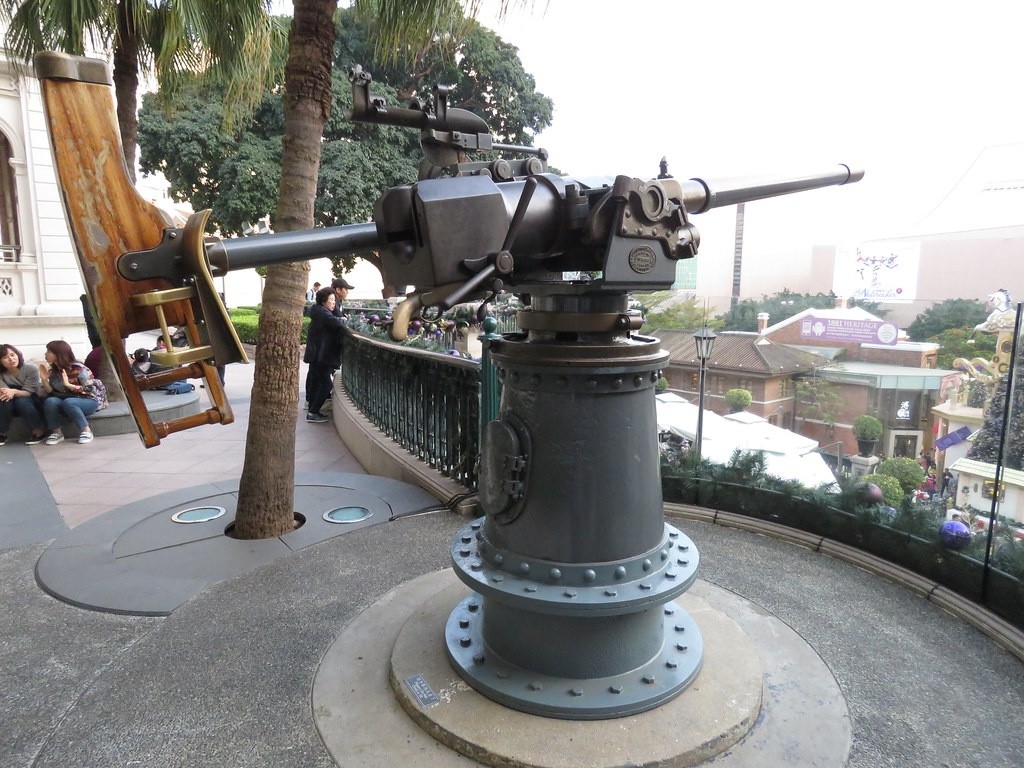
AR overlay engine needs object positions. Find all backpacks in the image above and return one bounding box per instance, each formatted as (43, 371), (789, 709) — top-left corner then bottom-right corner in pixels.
(67, 362), (109, 411)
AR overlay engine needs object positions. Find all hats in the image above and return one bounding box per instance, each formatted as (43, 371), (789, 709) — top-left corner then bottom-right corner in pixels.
(331, 278), (355, 289)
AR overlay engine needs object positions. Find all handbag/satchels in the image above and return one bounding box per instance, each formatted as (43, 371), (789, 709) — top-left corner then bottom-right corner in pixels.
(167, 381), (195, 395)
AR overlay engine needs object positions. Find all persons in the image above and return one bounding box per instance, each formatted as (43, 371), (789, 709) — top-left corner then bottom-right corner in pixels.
(39, 340), (103, 444)
(302, 279), (355, 409)
(0, 344), (50, 446)
(917, 450), (953, 503)
(305, 282), (321, 301)
(304, 287), (348, 422)
(80, 293), (227, 401)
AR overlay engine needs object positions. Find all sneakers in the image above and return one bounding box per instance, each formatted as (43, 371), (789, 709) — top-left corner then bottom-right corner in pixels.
(45, 432), (64, 444)
(77, 431), (94, 443)
(0, 434), (8, 445)
(306, 410), (329, 423)
(24, 431), (48, 445)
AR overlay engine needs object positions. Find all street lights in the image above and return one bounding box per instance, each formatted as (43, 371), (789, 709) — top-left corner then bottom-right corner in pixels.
(692, 326), (717, 460)
